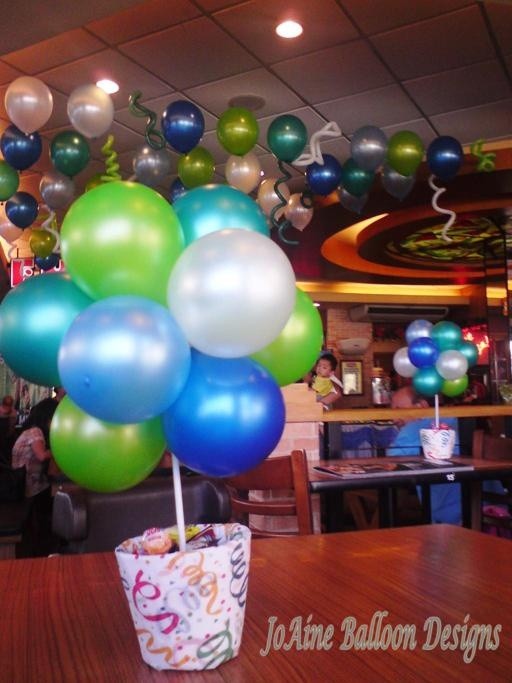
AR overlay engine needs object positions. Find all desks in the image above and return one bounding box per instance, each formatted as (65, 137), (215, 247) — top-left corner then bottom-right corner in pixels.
(307, 453), (512, 531)
(0, 523), (512, 682)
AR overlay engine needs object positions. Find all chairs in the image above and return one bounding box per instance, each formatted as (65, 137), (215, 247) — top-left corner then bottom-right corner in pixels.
(50, 476), (231, 554)
(473, 428), (512, 539)
(222, 448), (314, 538)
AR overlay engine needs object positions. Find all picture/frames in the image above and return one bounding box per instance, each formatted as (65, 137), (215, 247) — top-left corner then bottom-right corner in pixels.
(340, 359), (364, 396)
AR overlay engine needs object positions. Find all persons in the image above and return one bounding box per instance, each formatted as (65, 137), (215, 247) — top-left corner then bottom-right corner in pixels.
(310, 350), (346, 439)
(303, 351), (347, 460)
(53, 386), (67, 402)
(10, 396), (63, 553)
(460, 376), (492, 430)
(370, 384), (509, 530)
(0, 392), (20, 431)
(246, 365), (329, 536)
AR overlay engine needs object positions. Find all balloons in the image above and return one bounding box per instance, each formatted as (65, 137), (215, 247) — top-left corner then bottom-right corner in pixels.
(158, 347), (286, 480)
(166, 225), (298, 360)
(0, 273), (96, 390)
(392, 317), (479, 399)
(60, 178), (183, 305)
(54, 294), (191, 425)
(3, 70), (468, 275)
(248, 286), (327, 389)
(47, 393), (169, 494)
